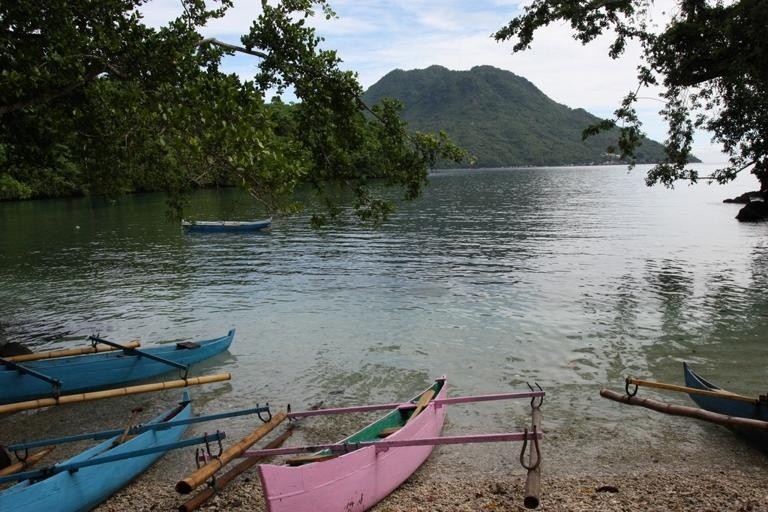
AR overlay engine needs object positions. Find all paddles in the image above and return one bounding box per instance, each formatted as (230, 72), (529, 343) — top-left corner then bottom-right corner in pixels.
(407, 389), (435, 422)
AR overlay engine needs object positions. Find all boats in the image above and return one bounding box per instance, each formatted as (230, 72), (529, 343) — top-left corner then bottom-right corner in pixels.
(181, 217), (273, 229)
(183, 227), (274, 238)
(0, 327), (235, 402)
(257, 373), (452, 511)
(683, 360), (768, 445)
(0, 389), (196, 512)
